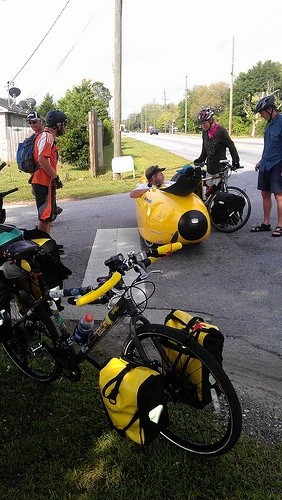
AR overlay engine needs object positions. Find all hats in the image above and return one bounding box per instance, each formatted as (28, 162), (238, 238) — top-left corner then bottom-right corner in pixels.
(26, 112), (41, 122)
(145, 165), (166, 179)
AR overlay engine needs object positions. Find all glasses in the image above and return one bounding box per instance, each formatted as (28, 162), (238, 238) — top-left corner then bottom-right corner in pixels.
(28, 120), (38, 125)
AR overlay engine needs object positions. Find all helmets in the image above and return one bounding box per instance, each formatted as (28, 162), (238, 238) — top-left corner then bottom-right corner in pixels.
(256, 94), (275, 112)
(197, 108), (215, 122)
(46, 110), (68, 126)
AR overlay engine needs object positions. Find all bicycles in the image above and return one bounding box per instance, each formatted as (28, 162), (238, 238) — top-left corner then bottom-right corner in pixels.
(174, 159), (252, 233)
(1, 239), (243, 460)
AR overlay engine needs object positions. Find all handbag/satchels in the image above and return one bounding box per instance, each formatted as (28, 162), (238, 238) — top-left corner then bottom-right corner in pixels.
(160, 309), (223, 410)
(17, 230), (71, 319)
(98, 358), (172, 446)
(213, 192), (246, 226)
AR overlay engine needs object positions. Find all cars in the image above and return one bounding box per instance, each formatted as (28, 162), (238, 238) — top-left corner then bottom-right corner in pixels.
(149, 128), (158, 135)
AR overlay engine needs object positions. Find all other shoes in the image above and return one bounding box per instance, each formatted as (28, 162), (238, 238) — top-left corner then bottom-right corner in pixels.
(50, 206), (63, 221)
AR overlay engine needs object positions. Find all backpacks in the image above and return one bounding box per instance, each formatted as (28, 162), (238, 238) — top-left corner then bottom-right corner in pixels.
(17, 130), (54, 173)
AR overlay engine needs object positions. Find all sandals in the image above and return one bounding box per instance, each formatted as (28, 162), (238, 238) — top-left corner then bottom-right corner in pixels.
(250, 222), (271, 231)
(272, 226), (282, 236)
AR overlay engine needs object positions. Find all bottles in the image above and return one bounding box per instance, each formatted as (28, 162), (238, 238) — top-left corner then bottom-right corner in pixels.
(205, 183), (216, 197)
(202, 184), (207, 201)
(73, 314), (94, 344)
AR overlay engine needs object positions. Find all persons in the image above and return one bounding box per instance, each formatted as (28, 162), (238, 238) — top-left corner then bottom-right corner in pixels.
(129, 166), (177, 198)
(194, 108), (240, 228)
(251, 95), (282, 237)
(26, 109), (68, 254)
